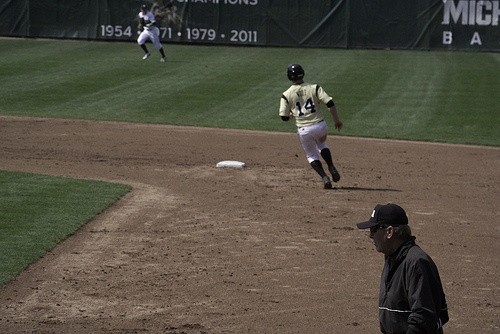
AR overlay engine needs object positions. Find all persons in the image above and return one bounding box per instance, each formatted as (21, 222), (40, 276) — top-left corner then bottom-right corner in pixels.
(357, 202), (449, 334)
(137, 4), (166, 63)
(279, 64), (343, 189)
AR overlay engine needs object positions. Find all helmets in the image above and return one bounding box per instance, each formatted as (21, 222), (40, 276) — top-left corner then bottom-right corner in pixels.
(287, 64), (304, 80)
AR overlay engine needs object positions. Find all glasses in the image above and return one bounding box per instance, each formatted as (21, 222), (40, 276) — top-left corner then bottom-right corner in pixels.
(370, 226), (400, 234)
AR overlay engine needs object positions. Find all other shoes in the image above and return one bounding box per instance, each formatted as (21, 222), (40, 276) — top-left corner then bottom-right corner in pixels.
(323, 176), (332, 189)
(142, 53), (151, 60)
(331, 169), (340, 183)
(160, 57), (166, 62)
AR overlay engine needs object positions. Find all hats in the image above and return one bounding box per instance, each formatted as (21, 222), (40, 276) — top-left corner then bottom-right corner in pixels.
(140, 5), (147, 8)
(357, 202), (408, 229)
